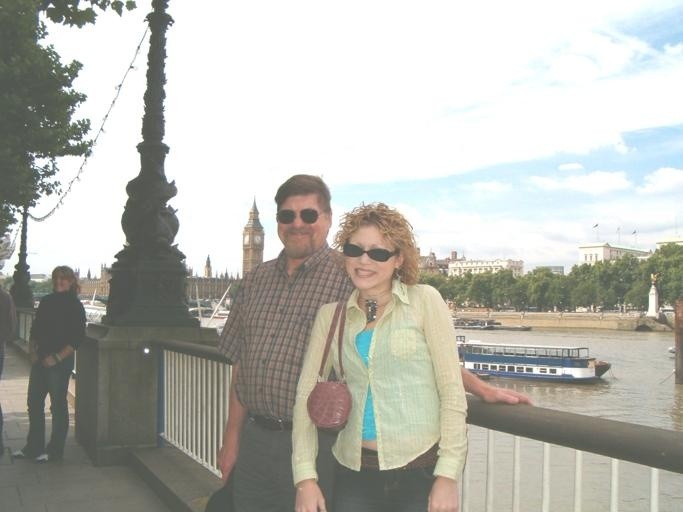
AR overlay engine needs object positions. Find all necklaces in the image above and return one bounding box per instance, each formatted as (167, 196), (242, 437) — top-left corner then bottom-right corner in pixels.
(358, 290), (393, 322)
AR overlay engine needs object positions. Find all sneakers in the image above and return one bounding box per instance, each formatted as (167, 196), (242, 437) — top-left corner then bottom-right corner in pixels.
(11, 441), (63, 463)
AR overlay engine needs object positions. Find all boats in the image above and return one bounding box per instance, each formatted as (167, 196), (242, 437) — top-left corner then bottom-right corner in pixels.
(456, 335), (611, 383)
(453, 317), (532, 331)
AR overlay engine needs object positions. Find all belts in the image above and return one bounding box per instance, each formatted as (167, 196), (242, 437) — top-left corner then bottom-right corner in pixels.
(256, 418), (293, 431)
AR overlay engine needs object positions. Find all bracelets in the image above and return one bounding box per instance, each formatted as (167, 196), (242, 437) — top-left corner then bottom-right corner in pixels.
(53, 353), (63, 363)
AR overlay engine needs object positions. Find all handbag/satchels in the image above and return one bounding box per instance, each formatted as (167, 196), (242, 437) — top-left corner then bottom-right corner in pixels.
(307, 380), (352, 430)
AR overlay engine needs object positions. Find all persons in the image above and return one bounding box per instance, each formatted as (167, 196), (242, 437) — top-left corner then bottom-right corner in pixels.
(0, 289), (18, 456)
(204, 176), (533, 511)
(14, 264), (87, 462)
(288, 201), (470, 511)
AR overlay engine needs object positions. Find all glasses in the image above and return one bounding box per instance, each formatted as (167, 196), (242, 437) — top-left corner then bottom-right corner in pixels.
(276, 209), (318, 224)
(343, 238), (396, 261)
